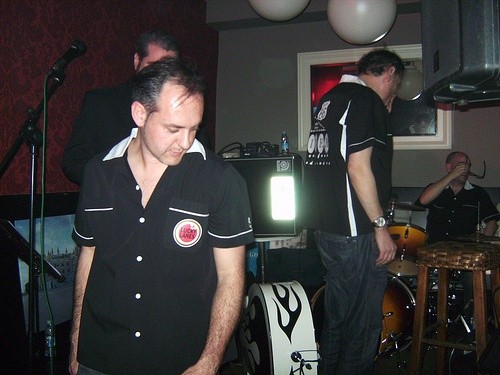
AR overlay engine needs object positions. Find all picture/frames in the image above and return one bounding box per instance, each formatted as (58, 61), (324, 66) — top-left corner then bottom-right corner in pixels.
(296, 44), (452, 155)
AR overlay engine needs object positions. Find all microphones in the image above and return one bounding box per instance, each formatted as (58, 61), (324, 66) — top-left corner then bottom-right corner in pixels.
(46, 37), (89, 78)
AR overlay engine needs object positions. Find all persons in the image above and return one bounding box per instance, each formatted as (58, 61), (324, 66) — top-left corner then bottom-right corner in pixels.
(413, 151), (500, 324)
(68, 58), (255, 375)
(402, 112), (432, 135)
(61, 30), (180, 185)
(301, 49), (406, 375)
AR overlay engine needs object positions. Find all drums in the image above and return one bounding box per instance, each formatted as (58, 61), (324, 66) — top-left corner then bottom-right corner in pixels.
(312, 274), (417, 360)
(385, 222), (428, 277)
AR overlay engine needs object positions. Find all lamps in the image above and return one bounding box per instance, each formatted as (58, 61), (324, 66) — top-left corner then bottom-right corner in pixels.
(249, 0), (309, 21)
(327, 0), (397, 45)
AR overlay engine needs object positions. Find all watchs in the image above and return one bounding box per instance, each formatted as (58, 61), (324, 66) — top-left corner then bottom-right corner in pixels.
(372, 216), (386, 228)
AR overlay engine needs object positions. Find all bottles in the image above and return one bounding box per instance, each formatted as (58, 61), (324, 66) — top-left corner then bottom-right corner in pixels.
(44, 319), (57, 359)
(281, 132), (289, 156)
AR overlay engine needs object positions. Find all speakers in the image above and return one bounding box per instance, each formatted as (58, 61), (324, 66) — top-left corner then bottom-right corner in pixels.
(220, 154), (305, 236)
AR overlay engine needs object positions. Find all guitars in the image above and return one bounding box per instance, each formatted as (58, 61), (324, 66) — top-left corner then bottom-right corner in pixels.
(237, 281), (318, 375)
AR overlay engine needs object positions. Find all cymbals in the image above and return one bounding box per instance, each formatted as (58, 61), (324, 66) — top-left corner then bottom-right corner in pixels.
(388, 201), (426, 210)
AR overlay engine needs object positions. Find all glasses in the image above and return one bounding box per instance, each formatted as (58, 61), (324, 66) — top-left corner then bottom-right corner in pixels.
(465, 156), (487, 179)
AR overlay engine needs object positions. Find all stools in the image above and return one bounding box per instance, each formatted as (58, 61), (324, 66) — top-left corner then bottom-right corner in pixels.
(409, 239), (500, 375)
